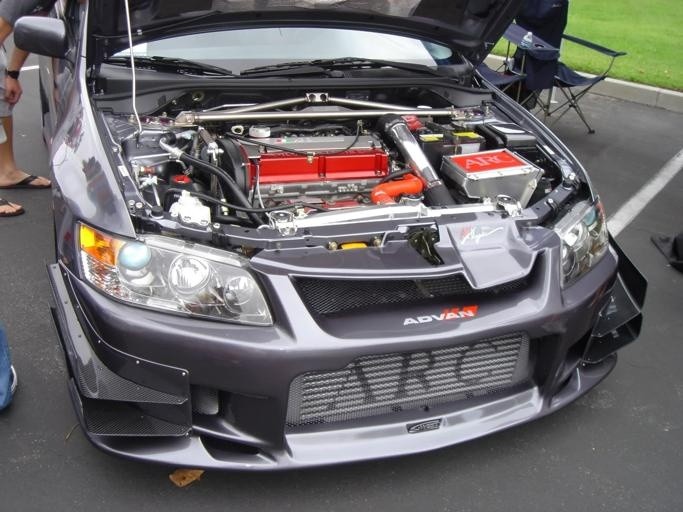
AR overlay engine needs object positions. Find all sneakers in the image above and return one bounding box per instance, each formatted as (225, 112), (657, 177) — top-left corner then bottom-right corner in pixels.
(10, 365), (18, 395)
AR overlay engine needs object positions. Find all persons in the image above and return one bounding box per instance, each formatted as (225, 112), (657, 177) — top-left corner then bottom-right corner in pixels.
(0, 320), (19, 413)
(0, 0), (58, 218)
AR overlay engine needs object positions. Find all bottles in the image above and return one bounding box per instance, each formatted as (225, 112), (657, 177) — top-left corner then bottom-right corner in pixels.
(521, 32), (534, 48)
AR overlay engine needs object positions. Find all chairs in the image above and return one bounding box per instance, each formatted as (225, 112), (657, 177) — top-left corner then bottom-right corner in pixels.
(476, 0), (627, 134)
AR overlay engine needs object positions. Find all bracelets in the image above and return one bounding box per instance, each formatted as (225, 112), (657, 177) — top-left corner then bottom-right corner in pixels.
(4, 68), (21, 80)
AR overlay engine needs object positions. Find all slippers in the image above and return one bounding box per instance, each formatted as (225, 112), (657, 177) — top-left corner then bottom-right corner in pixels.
(0, 176), (51, 189)
(0, 199), (25, 217)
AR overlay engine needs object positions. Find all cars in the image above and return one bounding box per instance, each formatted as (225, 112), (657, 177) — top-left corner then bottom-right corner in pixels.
(14, 0), (649, 472)
